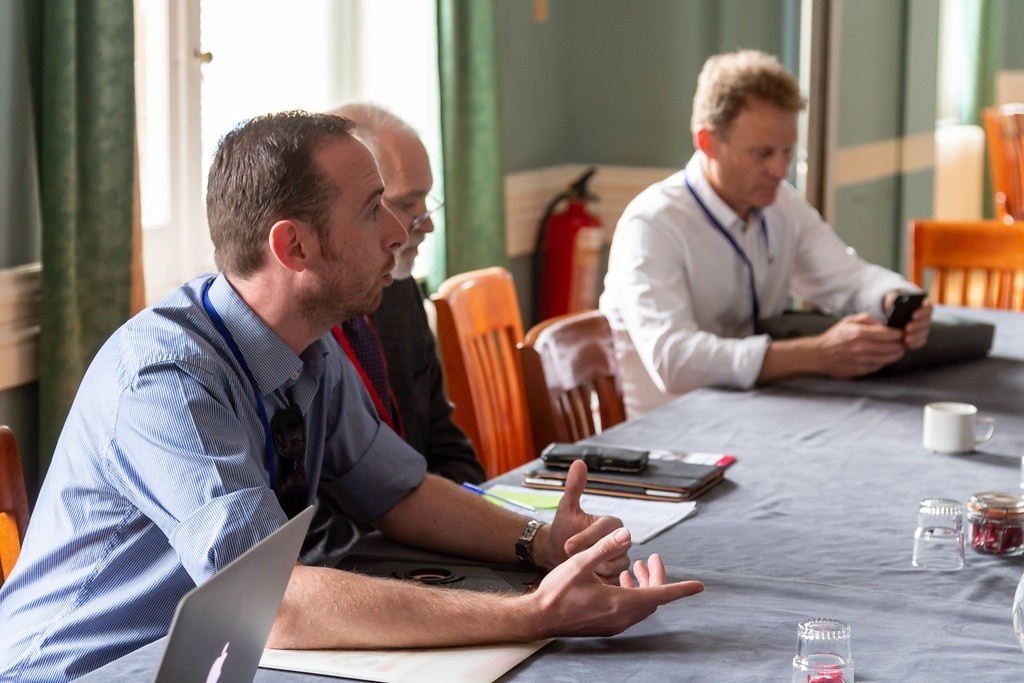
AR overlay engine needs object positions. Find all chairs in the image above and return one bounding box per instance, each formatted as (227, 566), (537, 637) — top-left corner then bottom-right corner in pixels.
(432, 269), (533, 482)
(517, 311), (628, 455)
(985, 99), (1024, 221)
(910, 218), (1024, 313)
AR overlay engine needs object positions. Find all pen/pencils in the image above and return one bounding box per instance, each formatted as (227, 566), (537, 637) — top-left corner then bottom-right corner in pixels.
(463, 481), (535, 513)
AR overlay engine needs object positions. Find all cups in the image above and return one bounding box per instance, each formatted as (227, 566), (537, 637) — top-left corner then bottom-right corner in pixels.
(923, 402), (996, 454)
(911, 499), (966, 573)
(965, 492), (1024, 558)
(790, 620), (855, 683)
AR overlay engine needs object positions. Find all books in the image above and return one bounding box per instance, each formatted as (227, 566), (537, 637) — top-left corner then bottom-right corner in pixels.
(517, 436), (726, 503)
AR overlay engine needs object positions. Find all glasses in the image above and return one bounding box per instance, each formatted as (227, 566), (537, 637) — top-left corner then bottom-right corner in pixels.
(270, 402), (311, 520)
(383, 193), (446, 232)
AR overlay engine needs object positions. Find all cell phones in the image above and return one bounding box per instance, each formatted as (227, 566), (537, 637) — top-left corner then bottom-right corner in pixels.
(884, 293), (926, 329)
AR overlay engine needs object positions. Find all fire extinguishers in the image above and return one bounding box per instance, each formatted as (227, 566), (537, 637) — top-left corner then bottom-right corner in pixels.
(527, 165), (605, 324)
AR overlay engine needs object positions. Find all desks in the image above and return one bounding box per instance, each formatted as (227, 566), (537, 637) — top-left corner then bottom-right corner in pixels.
(71, 305), (1024, 683)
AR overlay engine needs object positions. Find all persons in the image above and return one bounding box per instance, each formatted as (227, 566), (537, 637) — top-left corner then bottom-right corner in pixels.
(595, 46), (934, 417)
(339, 106), (485, 482)
(0, 111), (709, 683)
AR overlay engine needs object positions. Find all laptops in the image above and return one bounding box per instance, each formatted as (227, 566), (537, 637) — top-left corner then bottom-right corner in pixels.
(153, 505), (316, 683)
